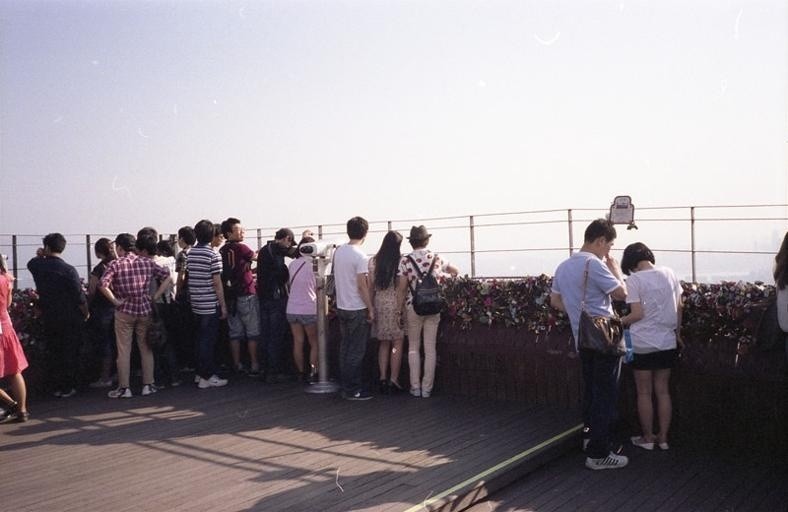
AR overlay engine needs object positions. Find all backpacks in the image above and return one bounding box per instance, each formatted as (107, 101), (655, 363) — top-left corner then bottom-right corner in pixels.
(223, 267), (247, 297)
(404, 253), (442, 316)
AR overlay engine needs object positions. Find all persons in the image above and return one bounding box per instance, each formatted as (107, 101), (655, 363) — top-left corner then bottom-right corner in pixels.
(80, 217), (319, 397)
(615, 241), (682, 455)
(1, 257), (29, 423)
(774, 229), (788, 352)
(332, 216), (458, 400)
(28, 232), (90, 397)
(548, 217), (628, 473)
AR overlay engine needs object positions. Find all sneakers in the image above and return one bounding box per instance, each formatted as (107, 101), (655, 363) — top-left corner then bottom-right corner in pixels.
(422, 390), (431, 397)
(409, 389), (421, 397)
(108, 387), (132, 398)
(194, 375), (228, 389)
(585, 451), (628, 470)
(142, 384), (158, 396)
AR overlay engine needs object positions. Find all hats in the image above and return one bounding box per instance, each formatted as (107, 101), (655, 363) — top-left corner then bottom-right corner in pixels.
(406, 225), (432, 240)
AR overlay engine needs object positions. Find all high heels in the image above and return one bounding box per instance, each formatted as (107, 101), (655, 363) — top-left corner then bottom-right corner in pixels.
(379, 379), (406, 396)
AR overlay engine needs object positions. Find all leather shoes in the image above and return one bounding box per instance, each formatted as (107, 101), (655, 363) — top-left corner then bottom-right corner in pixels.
(631, 436), (669, 450)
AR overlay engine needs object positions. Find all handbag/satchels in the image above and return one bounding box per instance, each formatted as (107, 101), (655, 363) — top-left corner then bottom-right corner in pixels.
(146, 320), (168, 349)
(577, 310), (627, 357)
(326, 274), (335, 297)
